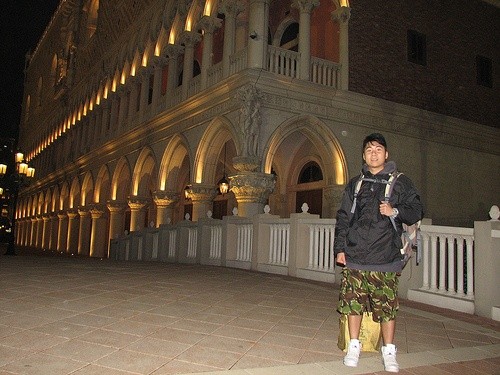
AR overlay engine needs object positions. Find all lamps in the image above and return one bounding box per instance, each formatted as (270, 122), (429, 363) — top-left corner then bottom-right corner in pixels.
(218, 143), (229, 195)
(184, 164), (191, 200)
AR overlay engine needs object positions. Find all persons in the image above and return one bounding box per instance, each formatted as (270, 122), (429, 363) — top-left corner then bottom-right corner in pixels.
(334, 133), (423, 373)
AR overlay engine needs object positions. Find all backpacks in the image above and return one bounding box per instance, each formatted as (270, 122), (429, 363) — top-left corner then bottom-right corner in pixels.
(350, 170), (422, 276)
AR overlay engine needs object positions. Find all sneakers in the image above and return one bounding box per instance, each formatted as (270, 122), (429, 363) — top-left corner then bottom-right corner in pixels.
(381, 344), (399, 373)
(344, 339), (362, 367)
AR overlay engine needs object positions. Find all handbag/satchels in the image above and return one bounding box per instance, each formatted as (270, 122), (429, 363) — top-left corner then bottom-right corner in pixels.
(337, 305), (381, 354)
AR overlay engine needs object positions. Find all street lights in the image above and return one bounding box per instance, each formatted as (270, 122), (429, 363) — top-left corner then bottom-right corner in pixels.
(0, 146), (37, 256)
